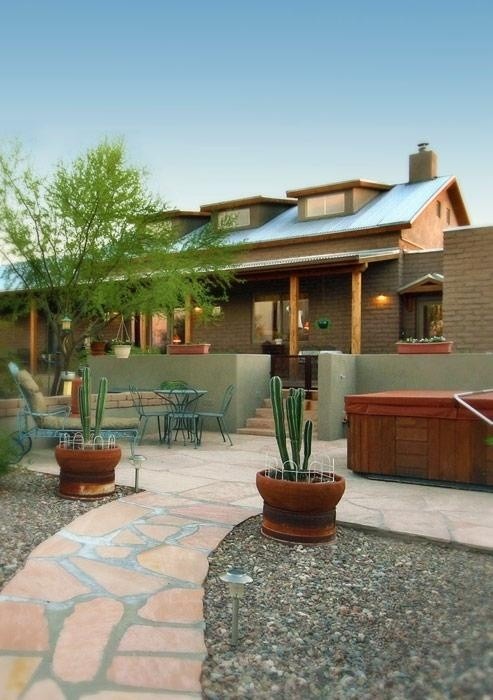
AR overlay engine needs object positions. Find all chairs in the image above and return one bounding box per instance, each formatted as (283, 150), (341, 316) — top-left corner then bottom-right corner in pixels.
(8, 358), (139, 467)
(127, 378), (237, 449)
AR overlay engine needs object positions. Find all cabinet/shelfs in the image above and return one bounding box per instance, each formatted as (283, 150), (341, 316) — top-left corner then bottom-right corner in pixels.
(342, 388), (492, 487)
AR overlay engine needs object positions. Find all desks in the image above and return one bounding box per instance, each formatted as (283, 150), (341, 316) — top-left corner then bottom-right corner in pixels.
(288, 351), (343, 397)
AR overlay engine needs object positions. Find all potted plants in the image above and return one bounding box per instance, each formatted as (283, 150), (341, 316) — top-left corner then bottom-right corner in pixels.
(165, 342), (211, 354)
(255, 373), (347, 546)
(111, 337), (133, 359)
(51, 364), (123, 502)
(394, 335), (456, 355)
(90, 334), (109, 355)
